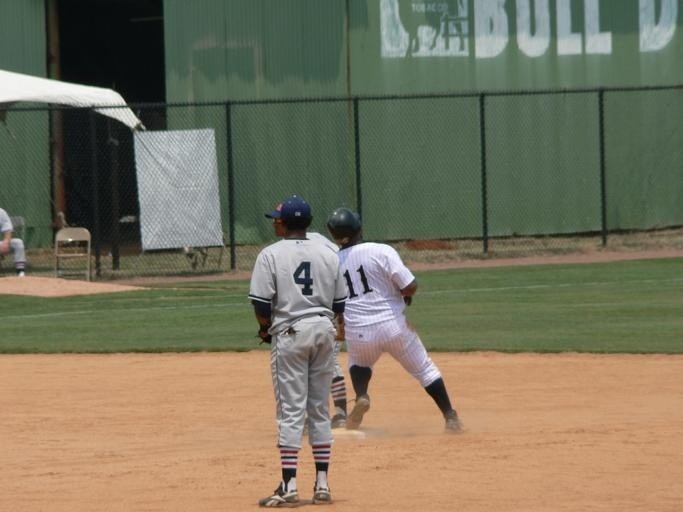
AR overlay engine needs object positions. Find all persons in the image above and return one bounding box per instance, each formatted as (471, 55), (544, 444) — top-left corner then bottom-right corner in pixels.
(264, 204), (347, 436)
(248, 197), (347, 508)
(0, 208), (27, 277)
(327, 207), (467, 434)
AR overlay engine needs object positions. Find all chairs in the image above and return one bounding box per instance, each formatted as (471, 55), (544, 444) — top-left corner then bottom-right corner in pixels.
(54, 227), (91, 282)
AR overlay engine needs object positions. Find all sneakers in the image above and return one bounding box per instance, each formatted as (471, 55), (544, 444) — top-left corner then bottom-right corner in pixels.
(330, 414), (346, 428)
(347, 394), (370, 428)
(313, 482), (331, 504)
(260, 485), (299, 507)
(446, 419), (463, 433)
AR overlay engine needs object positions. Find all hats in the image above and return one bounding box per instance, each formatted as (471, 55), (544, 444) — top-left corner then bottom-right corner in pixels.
(265, 194), (312, 229)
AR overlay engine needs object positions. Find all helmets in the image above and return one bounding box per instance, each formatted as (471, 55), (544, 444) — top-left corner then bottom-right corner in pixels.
(328, 208), (360, 235)
